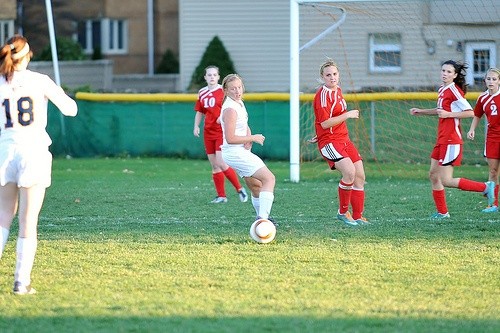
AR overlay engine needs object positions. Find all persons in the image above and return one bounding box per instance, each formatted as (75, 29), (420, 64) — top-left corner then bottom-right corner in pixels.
(193, 65), (248, 203)
(308, 57), (372, 226)
(0, 34), (77, 296)
(410, 59), (498, 220)
(467, 67), (500, 212)
(220, 73), (276, 243)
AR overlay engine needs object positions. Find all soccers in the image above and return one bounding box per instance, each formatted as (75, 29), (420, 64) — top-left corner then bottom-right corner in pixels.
(249, 218), (277, 245)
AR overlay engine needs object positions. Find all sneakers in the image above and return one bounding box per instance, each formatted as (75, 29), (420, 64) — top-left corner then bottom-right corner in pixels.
(268, 215), (279, 227)
(481, 206), (500, 213)
(256, 216), (261, 220)
(209, 197), (228, 204)
(337, 209), (358, 226)
(13, 281), (39, 295)
(483, 182), (495, 207)
(239, 187), (248, 203)
(430, 212), (450, 220)
(356, 216), (374, 226)
